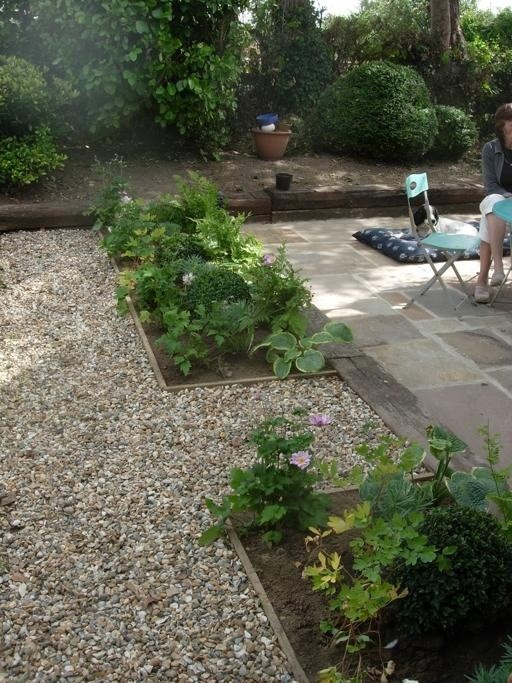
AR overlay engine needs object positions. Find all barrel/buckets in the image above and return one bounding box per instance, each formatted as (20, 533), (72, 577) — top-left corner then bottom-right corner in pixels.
(256, 112), (279, 132)
(276, 172), (292, 190)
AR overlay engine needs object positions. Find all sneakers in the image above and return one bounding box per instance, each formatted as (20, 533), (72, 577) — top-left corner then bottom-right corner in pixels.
(474, 285), (490, 304)
(490, 272), (506, 287)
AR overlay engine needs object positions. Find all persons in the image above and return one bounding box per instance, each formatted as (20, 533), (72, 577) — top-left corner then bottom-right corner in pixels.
(469, 102), (511, 304)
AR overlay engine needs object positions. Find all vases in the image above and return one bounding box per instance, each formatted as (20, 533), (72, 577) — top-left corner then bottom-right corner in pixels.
(250, 114), (292, 161)
(276, 173), (291, 190)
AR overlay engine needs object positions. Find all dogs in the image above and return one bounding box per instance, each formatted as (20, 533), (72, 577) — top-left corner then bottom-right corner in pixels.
(394, 204), (479, 249)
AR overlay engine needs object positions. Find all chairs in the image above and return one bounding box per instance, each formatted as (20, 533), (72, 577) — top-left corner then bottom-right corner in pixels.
(398, 172), (477, 322)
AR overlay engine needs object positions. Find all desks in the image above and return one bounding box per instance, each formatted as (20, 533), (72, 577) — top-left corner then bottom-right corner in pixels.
(485, 196), (511, 309)
